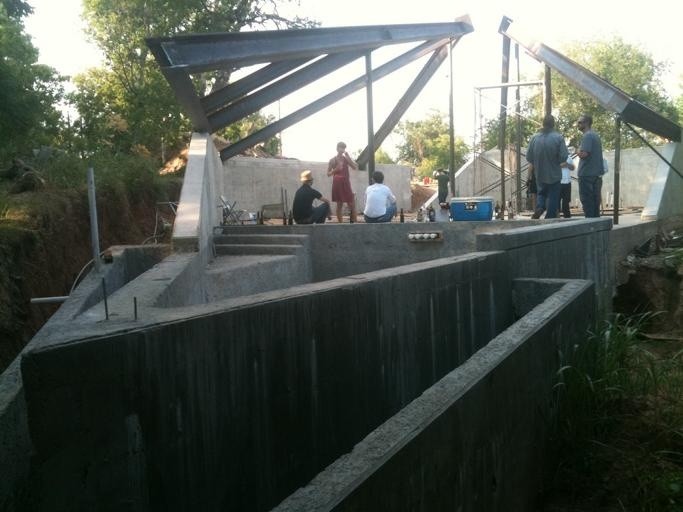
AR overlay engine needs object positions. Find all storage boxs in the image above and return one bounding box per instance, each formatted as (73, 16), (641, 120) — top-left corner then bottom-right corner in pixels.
(449, 196), (494, 222)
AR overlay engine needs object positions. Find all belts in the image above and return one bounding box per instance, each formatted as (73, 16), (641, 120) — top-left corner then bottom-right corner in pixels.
(365, 215), (384, 219)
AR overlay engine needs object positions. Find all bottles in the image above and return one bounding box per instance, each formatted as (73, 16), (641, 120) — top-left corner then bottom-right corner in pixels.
(259, 211), (263, 225)
(288, 210), (292, 225)
(400, 208), (403, 223)
(429, 203), (435, 222)
(416, 208), (424, 221)
(282, 212), (285, 225)
(256, 212), (260, 225)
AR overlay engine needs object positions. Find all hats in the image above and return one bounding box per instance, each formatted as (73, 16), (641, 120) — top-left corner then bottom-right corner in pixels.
(300, 170), (312, 181)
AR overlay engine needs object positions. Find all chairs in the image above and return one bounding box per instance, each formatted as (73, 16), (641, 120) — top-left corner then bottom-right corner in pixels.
(217, 194), (246, 223)
(260, 189), (288, 224)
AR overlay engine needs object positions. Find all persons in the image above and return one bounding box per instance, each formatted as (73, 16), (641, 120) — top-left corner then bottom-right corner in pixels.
(327, 142), (359, 223)
(432, 168), (450, 205)
(525, 114), (569, 219)
(570, 114), (608, 218)
(363, 171), (398, 222)
(560, 155), (576, 218)
(526, 165), (537, 210)
(293, 170), (332, 224)
